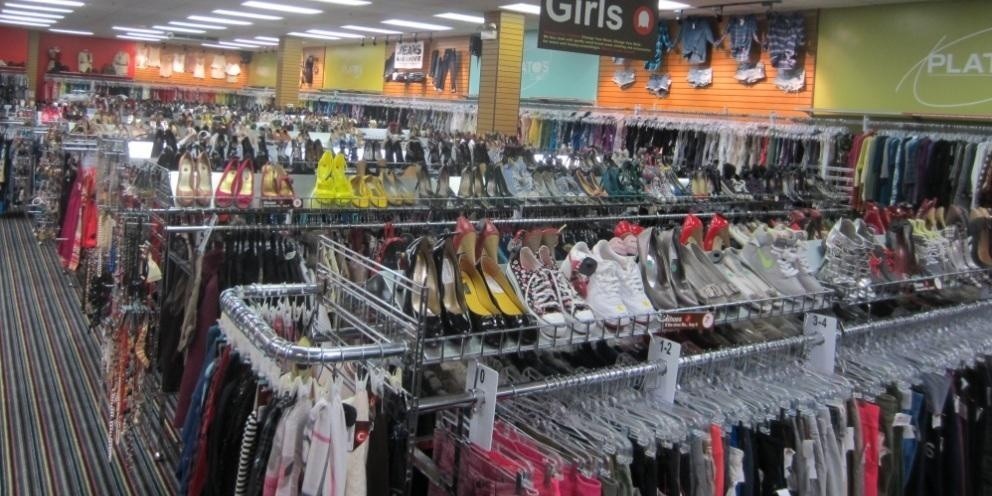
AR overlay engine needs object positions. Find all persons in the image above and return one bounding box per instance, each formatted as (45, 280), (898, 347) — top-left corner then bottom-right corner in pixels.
(114, 49), (129, 75)
(303, 55), (319, 88)
(77, 47), (93, 72)
(46, 44), (62, 72)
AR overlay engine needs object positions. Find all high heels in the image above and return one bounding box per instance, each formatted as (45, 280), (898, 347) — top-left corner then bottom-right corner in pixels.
(508, 225), (567, 264)
(658, 227), (701, 312)
(785, 208), (824, 240)
(483, 164), (525, 210)
(450, 215), (500, 265)
(862, 198), (945, 235)
(413, 165), (451, 211)
(814, 217), (878, 302)
(431, 235), (473, 347)
(396, 235), (445, 351)
(680, 212), (732, 253)
(632, 226), (679, 317)
(946, 204), (992, 278)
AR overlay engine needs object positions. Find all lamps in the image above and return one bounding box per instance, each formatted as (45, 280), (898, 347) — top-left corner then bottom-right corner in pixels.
(657, 0), (782, 25)
(360, 32), (433, 47)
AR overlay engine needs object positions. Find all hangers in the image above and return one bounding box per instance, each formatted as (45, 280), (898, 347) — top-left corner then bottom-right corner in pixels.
(862, 117), (991, 141)
(305, 93), (476, 127)
(419, 295), (992, 492)
(165, 191), (857, 283)
(516, 105), (848, 148)
(217, 283), (412, 406)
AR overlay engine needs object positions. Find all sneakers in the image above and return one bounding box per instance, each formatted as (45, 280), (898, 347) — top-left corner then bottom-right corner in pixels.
(741, 224), (825, 303)
(883, 218), (962, 291)
(559, 240), (632, 329)
(590, 238), (657, 324)
(534, 244), (597, 335)
(867, 243), (904, 295)
(505, 245), (568, 339)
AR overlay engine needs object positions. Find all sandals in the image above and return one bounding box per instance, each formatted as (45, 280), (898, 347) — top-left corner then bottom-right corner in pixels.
(314, 150), (354, 207)
(259, 161), (297, 208)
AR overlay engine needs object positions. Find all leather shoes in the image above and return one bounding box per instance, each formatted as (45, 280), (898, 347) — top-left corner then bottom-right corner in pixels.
(940, 225), (992, 289)
(213, 156), (255, 210)
(194, 150), (213, 207)
(706, 245), (787, 313)
(348, 170), (415, 209)
(176, 151), (195, 207)
(454, 252), (508, 349)
(475, 255), (539, 346)
(680, 243), (743, 312)
(457, 164), (490, 209)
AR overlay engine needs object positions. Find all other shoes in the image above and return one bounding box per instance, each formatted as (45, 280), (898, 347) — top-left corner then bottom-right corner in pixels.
(499, 142), (813, 206)
(150, 124), (496, 175)
